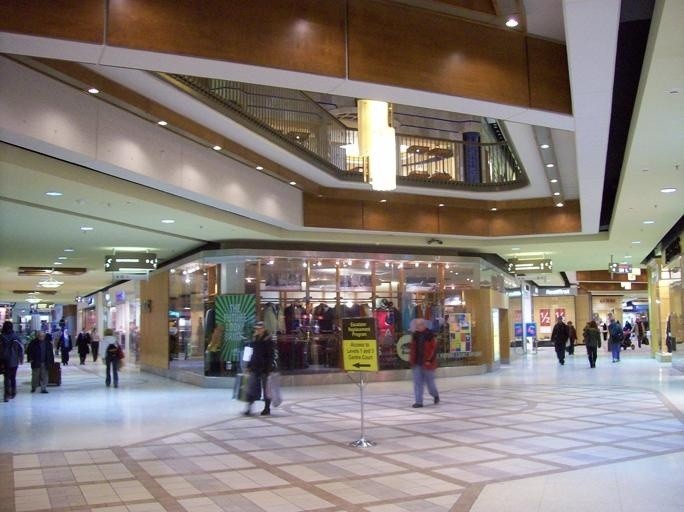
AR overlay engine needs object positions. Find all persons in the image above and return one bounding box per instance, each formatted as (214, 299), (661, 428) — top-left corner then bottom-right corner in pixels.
(548, 312), (650, 368)
(240, 321), (275, 414)
(0, 316), (125, 403)
(407, 317), (440, 408)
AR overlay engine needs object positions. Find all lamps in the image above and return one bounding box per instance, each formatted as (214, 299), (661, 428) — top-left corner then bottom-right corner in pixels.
(37, 277), (64, 288)
(356, 100), (398, 193)
(24, 294), (42, 304)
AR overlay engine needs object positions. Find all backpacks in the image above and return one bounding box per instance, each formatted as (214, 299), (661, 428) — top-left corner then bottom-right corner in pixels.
(107, 344), (117, 356)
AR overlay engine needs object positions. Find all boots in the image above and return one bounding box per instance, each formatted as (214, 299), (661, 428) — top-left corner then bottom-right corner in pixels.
(261, 399), (270, 415)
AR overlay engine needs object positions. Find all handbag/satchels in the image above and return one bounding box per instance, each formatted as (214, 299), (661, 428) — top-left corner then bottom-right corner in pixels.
(233, 374), (261, 401)
